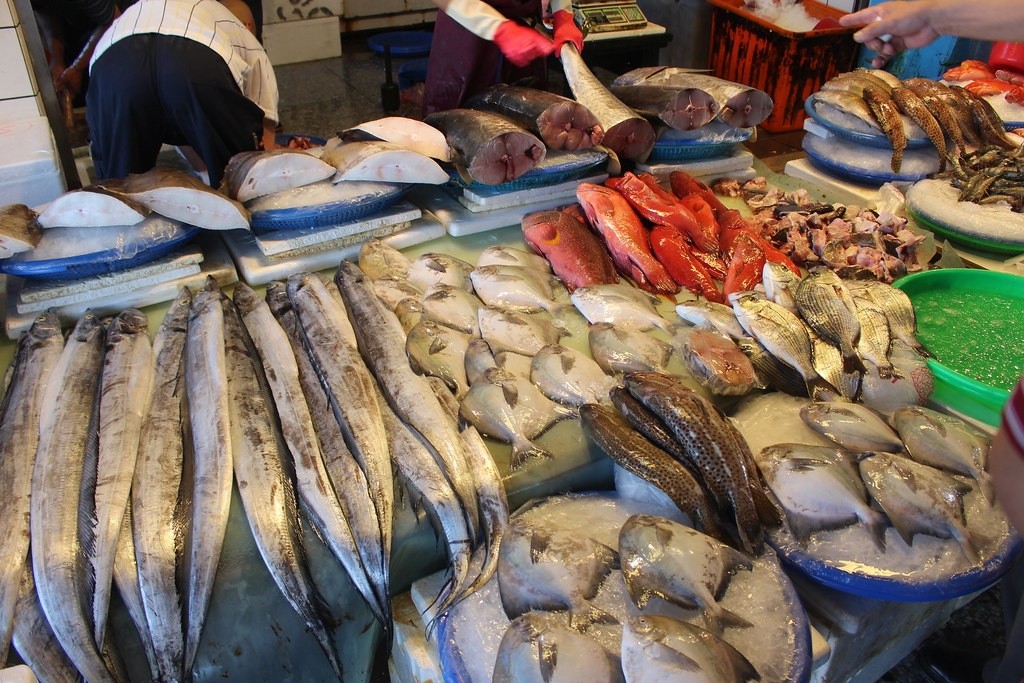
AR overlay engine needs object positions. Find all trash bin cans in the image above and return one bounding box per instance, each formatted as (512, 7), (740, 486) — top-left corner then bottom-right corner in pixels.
(707, 0), (867, 135)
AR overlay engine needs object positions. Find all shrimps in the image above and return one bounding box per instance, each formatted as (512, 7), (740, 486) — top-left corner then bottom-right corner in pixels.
(925, 145), (1024, 215)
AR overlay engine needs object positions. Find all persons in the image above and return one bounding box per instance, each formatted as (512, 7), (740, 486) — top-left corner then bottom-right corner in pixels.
(29, 0), (279, 188)
(422, 0), (584, 120)
(840, 0), (1024, 682)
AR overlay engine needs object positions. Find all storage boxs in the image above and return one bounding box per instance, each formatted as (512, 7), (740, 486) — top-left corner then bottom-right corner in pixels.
(262, 0), (344, 23)
(709, 0), (862, 133)
(261, 16), (342, 65)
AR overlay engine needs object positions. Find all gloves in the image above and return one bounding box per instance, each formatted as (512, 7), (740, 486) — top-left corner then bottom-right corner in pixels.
(549, 0), (585, 58)
(444, 0), (555, 69)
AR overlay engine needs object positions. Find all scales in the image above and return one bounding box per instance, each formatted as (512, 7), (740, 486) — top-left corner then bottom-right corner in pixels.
(572, 0), (648, 33)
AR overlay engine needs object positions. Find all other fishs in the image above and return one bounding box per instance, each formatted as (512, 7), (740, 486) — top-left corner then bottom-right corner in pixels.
(0, 16), (1010, 683)
